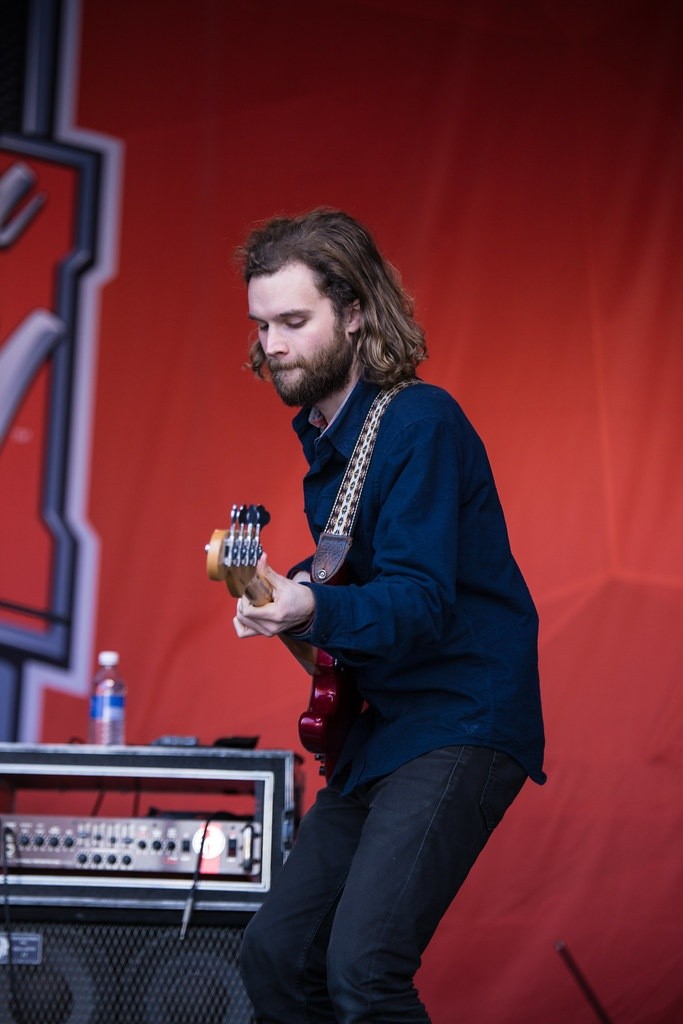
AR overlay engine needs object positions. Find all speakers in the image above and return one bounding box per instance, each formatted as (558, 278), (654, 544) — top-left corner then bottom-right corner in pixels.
(0, 896), (264, 1024)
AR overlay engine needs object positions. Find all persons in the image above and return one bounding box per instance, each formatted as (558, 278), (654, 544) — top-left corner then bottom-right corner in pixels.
(232, 209), (547, 1024)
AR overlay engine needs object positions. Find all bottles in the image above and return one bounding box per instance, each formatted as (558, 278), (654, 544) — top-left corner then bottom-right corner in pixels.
(88, 652), (124, 747)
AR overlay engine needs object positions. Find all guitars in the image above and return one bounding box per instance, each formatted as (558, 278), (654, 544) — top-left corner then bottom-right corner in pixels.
(203, 503), (363, 786)
